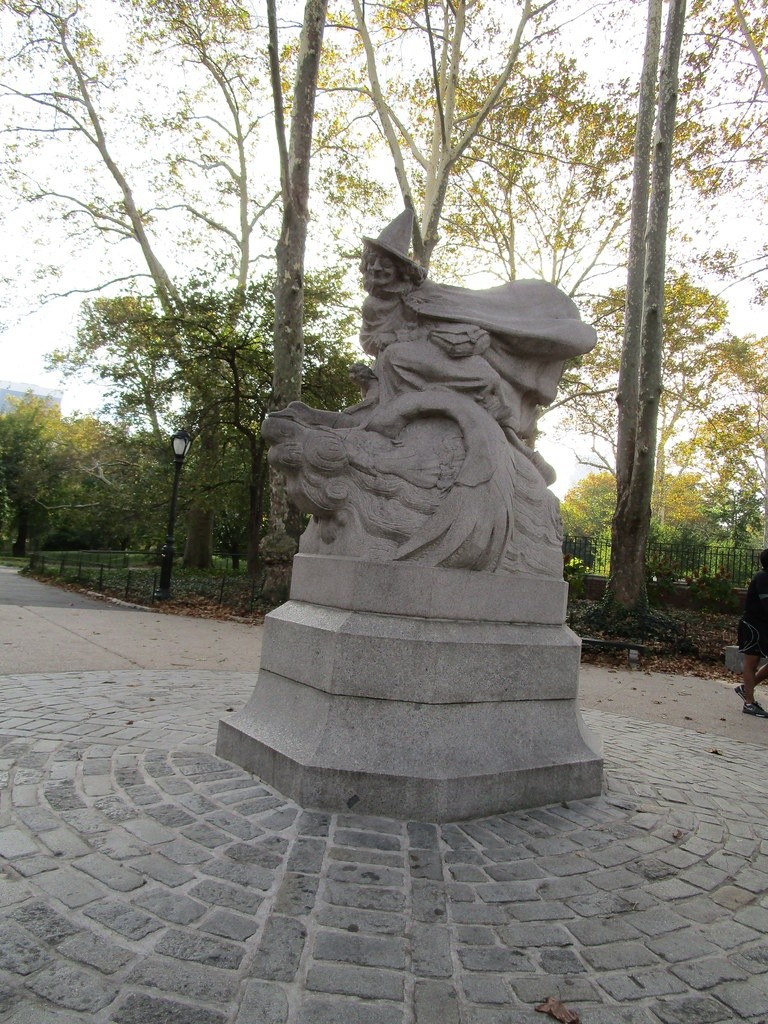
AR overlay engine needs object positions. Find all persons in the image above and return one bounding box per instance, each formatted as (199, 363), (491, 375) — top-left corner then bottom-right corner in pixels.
(733, 550), (768, 719)
(358, 235), (514, 425)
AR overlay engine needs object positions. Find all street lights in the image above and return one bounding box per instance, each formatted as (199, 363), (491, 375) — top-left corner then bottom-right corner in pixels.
(154, 426), (192, 602)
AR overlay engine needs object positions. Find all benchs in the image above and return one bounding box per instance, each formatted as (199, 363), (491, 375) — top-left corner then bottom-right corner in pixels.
(580, 638), (646, 668)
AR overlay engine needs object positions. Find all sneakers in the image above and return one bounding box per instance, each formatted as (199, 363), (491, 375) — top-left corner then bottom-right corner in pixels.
(734, 685), (746, 702)
(743, 701), (768, 719)
